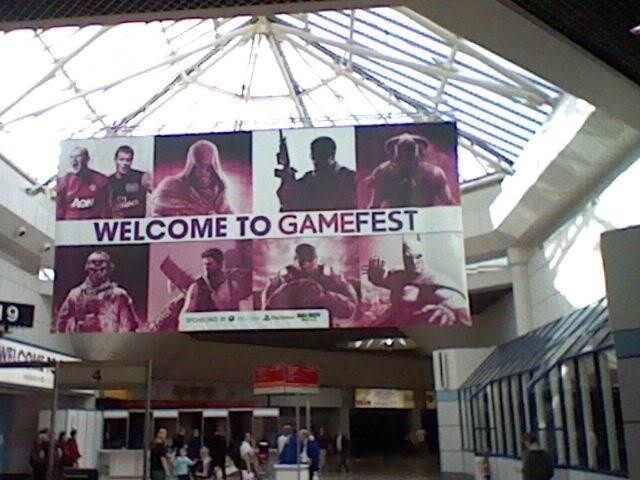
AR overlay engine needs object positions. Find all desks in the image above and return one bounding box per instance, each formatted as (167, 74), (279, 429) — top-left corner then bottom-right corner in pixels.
(273, 464), (310, 480)
(96, 449), (144, 480)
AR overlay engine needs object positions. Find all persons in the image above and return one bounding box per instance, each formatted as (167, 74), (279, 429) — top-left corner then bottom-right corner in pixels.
(56, 145), (153, 221)
(31, 426), (82, 480)
(153, 139), (232, 218)
(519, 432), (554, 480)
(364, 133), (454, 210)
(276, 136), (356, 212)
(480, 449), (492, 480)
(147, 423), (353, 480)
(56, 250), (141, 332)
(177, 239), (467, 330)
